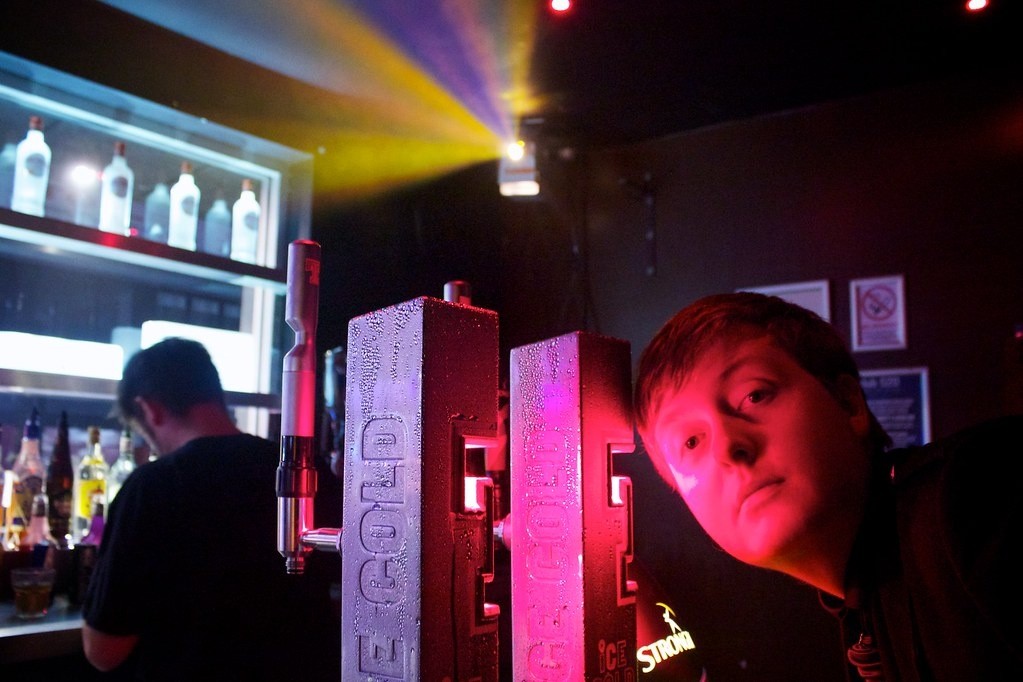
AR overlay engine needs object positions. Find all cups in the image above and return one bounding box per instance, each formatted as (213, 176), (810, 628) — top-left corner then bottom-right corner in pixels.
(11, 568), (55, 618)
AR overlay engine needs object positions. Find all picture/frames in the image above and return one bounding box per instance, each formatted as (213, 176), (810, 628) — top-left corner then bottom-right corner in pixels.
(849, 274), (908, 350)
(729, 281), (831, 323)
(855, 365), (933, 446)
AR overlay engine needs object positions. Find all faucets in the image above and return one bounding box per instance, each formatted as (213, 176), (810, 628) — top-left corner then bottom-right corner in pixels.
(271, 239), (343, 577)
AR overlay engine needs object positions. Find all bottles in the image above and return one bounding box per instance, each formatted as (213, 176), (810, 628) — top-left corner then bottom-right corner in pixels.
(204, 189), (232, 257)
(73, 426), (112, 537)
(46, 410), (74, 537)
(11, 117), (52, 218)
(110, 429), (138, 503)
(98, 143), (135, 237)
(167, 161), (202, 252)
(0, 130), (20, 210)
(230, 180), (264, 265)
(6, 407), (47, 543)
(143, 174), (170, 244)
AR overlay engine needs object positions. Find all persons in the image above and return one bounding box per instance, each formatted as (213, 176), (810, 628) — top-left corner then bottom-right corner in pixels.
(80, 338), (342, 682)
(630, 291), (1023, 682)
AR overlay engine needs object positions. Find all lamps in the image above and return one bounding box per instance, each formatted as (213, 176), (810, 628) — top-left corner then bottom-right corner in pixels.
(497, 154), (542, 198)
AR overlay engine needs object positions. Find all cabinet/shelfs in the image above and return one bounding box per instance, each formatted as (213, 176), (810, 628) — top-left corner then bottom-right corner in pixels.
(0, 53), (318, 682)
